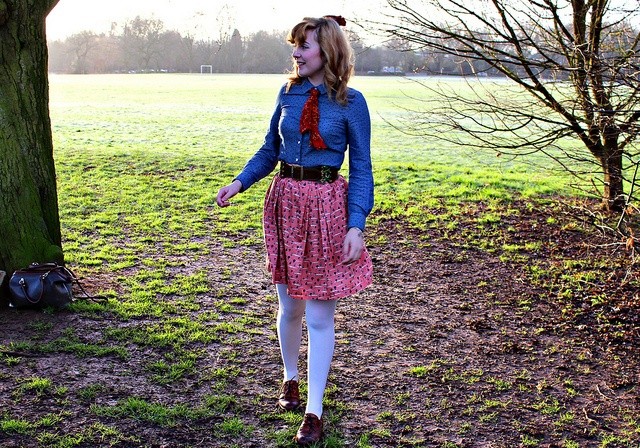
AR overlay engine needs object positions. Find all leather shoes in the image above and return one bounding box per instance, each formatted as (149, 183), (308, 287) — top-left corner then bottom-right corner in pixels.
(295, 413), (324, 446)
(279, 376), (301, 411)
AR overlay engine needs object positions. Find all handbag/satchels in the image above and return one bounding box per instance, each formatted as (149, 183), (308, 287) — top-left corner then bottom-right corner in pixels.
(8, 261), (108, 315)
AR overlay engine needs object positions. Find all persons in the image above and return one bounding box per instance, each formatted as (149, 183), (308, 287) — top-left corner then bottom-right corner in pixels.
(217, 15), (374, 444)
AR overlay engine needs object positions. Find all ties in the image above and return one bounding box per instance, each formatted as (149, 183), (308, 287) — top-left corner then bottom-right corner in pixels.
(299, 91), (326, 151)
